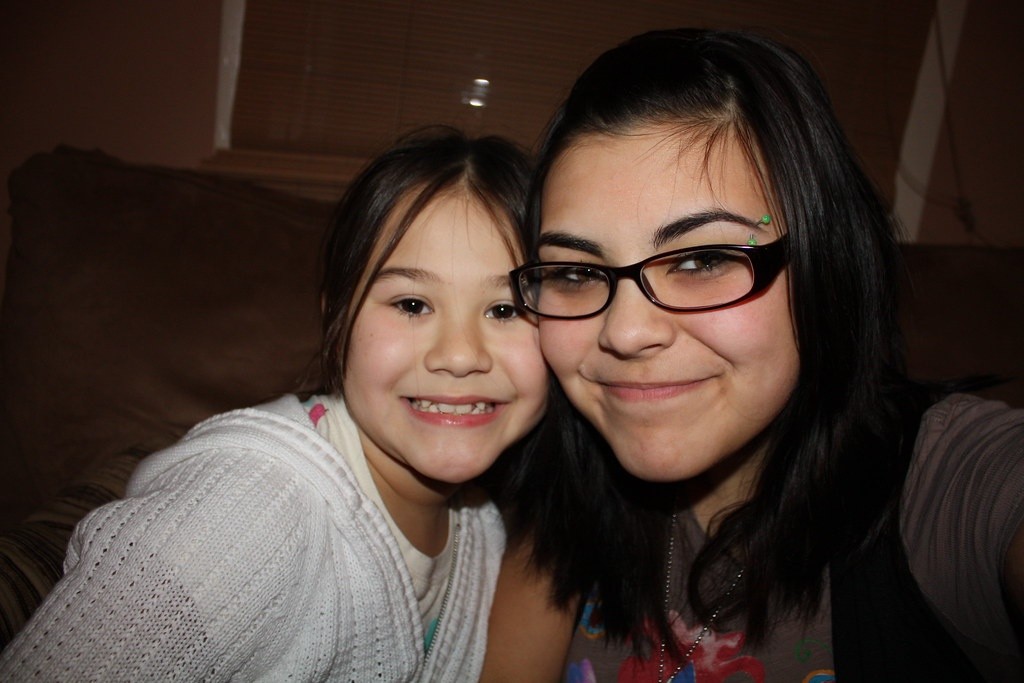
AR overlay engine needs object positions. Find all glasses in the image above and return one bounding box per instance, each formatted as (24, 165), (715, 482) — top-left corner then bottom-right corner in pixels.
(509, 235), (788, 322)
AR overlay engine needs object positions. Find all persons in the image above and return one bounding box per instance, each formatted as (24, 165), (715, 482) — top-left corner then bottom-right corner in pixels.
(0, 123), (549, 683)
(477, 27), (1024, 683)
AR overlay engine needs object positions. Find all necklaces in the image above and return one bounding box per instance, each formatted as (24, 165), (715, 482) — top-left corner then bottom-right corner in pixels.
(657, 477), (743, 683)
